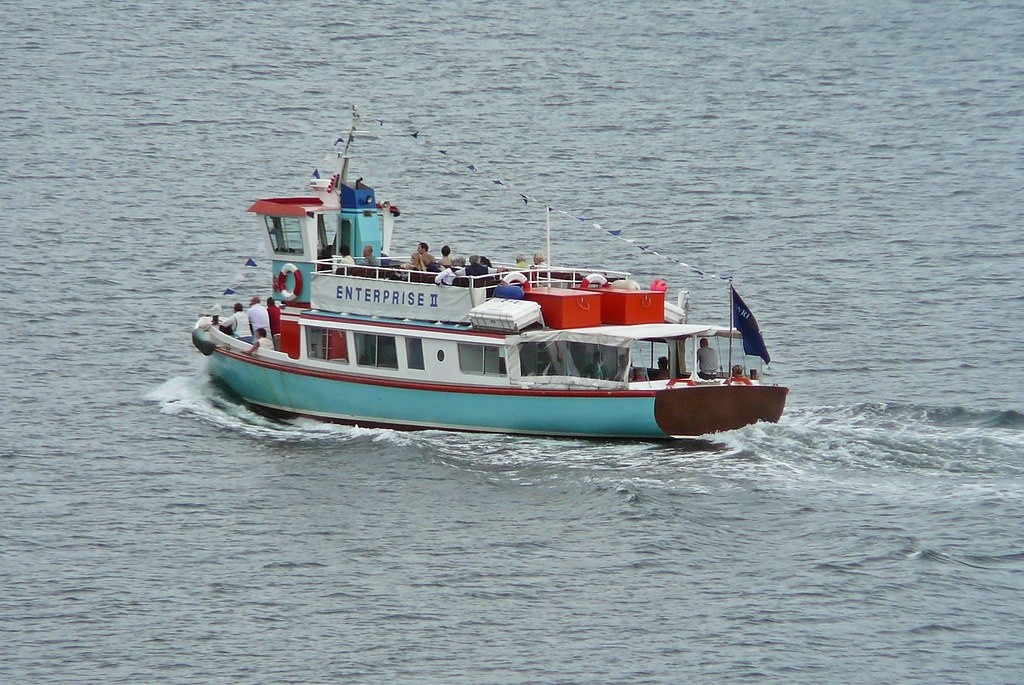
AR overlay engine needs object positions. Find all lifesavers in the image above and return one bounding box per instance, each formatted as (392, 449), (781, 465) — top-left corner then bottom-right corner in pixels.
(580, 273), (611, 288)
(278, 263), (303, 302)
(501, 273), (530, 290)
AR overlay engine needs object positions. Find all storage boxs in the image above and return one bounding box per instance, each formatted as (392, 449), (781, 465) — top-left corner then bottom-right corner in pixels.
(571, 288), (665, 324)
(529, 287), (603, 329)
(470, 298), (541, 331)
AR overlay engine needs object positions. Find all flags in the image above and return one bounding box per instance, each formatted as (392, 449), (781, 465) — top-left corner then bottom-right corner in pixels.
(732, 286), (770, 365)
(224, 288), (234, 295)
(313, 170), (320, 179)
(245, 259), (257, 267)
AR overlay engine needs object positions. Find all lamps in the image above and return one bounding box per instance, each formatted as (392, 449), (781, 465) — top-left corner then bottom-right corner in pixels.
(365, 195), (373, 203)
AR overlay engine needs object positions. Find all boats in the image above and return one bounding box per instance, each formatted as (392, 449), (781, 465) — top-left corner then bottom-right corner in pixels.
(193, 107), (790, 442)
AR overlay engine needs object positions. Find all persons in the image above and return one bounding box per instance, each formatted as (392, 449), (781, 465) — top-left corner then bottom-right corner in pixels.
(732, 365), (745, 378)
(697, 338), (718, 380)
(530, 251), (547, 269)
(588, 352), (646, 382)
(221, 297), (280, 354)
(393, 242), (509, 286)
(654, 357), (668, 380)
(337, 245), (355, 268)
(362, 246), (379, 266)
(514, 254), (529, 269)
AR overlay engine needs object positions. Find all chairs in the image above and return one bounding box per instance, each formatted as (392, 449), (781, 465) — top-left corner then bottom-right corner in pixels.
(335, 263), (583, 288)
